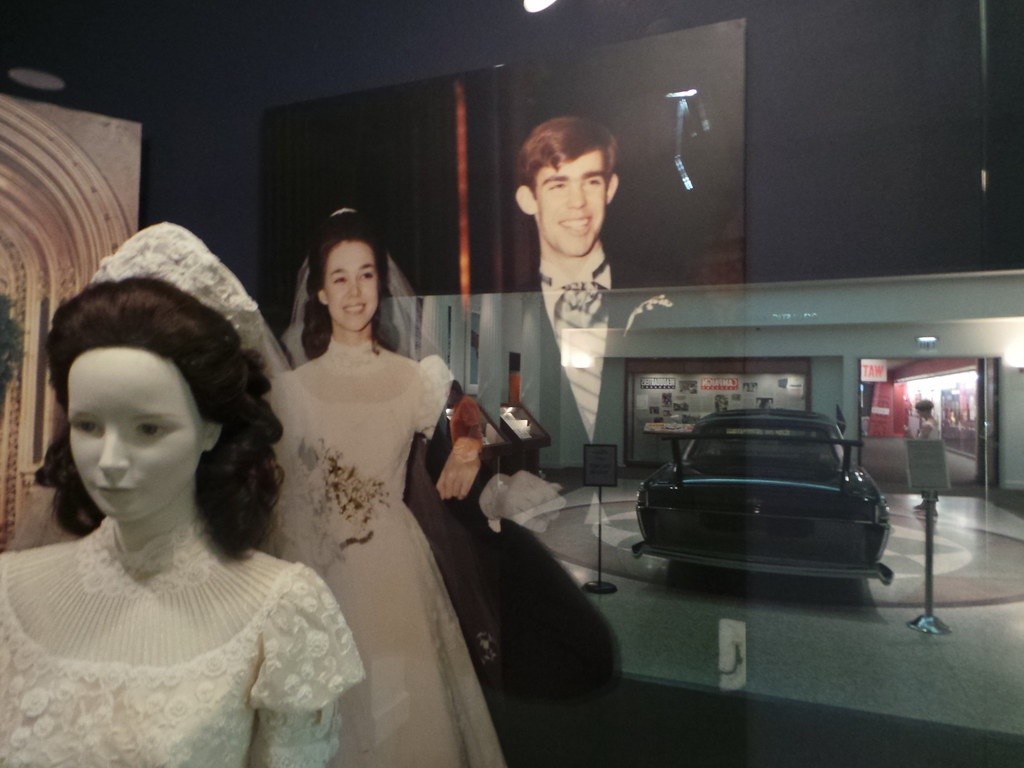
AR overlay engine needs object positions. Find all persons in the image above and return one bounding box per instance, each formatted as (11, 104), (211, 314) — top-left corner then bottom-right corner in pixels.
(430, 117), (688, 532)
(259, 205), (508, 768)
(904, 400), (940, 521)
(0, 220), (367, 768)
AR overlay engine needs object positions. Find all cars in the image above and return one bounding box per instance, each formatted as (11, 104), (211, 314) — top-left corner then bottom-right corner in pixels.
(629, 408), (897, 588)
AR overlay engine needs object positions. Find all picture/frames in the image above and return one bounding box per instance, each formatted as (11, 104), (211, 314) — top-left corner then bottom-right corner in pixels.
(409, 297), (424, 363)
(464, 311), (481, 392)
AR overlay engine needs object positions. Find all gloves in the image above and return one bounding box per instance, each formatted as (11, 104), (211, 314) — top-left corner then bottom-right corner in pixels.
(479, 471), (566, 533)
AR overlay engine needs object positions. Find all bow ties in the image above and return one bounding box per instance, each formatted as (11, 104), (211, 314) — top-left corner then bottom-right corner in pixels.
(539, 258), (609, 324)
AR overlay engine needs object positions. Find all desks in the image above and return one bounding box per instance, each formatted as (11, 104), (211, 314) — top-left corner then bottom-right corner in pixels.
(643, 422), (789, 435)
